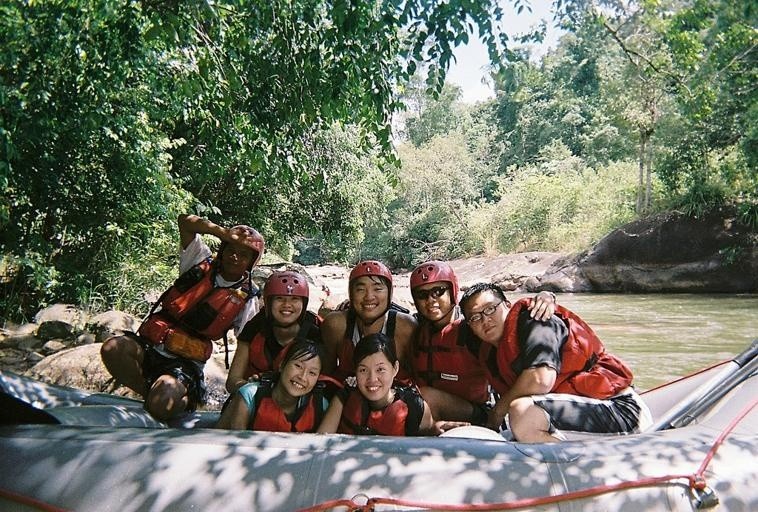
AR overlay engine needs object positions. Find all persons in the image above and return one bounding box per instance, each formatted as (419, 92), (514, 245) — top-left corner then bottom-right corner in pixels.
(214, 334), (344, 436)
(315, 332), (471, 438)
(225, 268), (324, 394)
(316, 259), (419, 386)
(403, 260), (496, 428)
(458, 283), (653, 443)
(101, 214), (265, 424)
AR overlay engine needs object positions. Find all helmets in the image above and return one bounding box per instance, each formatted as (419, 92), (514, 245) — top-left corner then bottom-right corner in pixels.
(217, 224), (264, 253)
(263, 271), (310, 299)
(348, 260), (392, 286)
(410, 261), (458, 289)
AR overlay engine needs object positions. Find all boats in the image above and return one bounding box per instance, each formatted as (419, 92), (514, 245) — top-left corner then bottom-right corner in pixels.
(0, 339), (757, 511)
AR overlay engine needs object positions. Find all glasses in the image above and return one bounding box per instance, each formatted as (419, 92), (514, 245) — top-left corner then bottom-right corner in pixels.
(414, 285), (450, 300)
(465, 300), (504, 324)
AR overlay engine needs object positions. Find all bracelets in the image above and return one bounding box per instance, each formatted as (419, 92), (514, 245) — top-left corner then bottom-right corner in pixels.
(550, 291), (557, 304)
